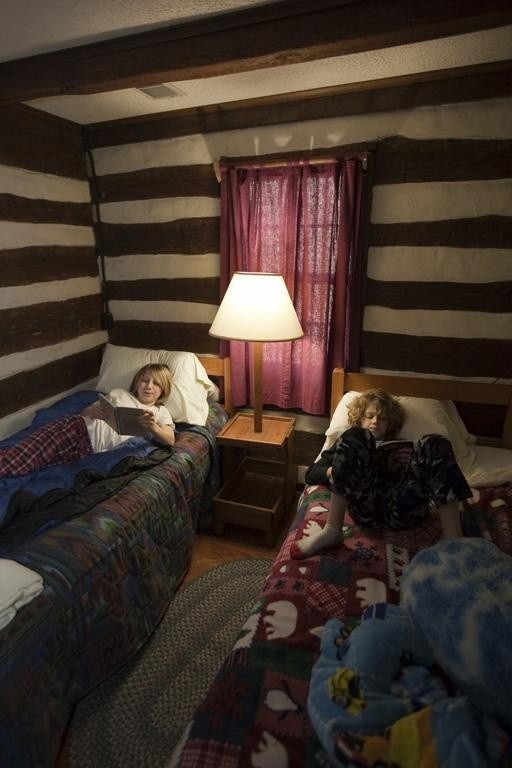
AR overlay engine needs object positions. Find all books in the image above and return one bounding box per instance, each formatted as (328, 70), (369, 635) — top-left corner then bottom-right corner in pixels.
(376, 438), (413, 479)
(99, 393), (152, 439)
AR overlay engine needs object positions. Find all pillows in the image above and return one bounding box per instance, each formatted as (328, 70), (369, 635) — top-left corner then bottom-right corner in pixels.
(93, 343), (211, 428)
(314, 392), (475, 474)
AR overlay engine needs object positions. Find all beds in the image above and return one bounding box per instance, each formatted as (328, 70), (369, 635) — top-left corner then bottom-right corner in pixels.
(0, 344), (228, 768)
(183, 366), (511, 767)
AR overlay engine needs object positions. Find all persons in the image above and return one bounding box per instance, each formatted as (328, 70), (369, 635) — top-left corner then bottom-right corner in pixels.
(0, 363), (175, 478)
(289, 387), (473, 561)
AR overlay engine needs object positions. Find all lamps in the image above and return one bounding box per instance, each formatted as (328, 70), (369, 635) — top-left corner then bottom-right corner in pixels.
(207, 269), (304, 432)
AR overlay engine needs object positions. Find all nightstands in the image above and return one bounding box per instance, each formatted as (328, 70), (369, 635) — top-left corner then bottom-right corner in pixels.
(211, 412), (299, 543)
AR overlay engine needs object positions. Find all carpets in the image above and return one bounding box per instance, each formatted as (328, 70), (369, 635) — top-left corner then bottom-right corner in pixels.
(59, 558), (276, 768)
